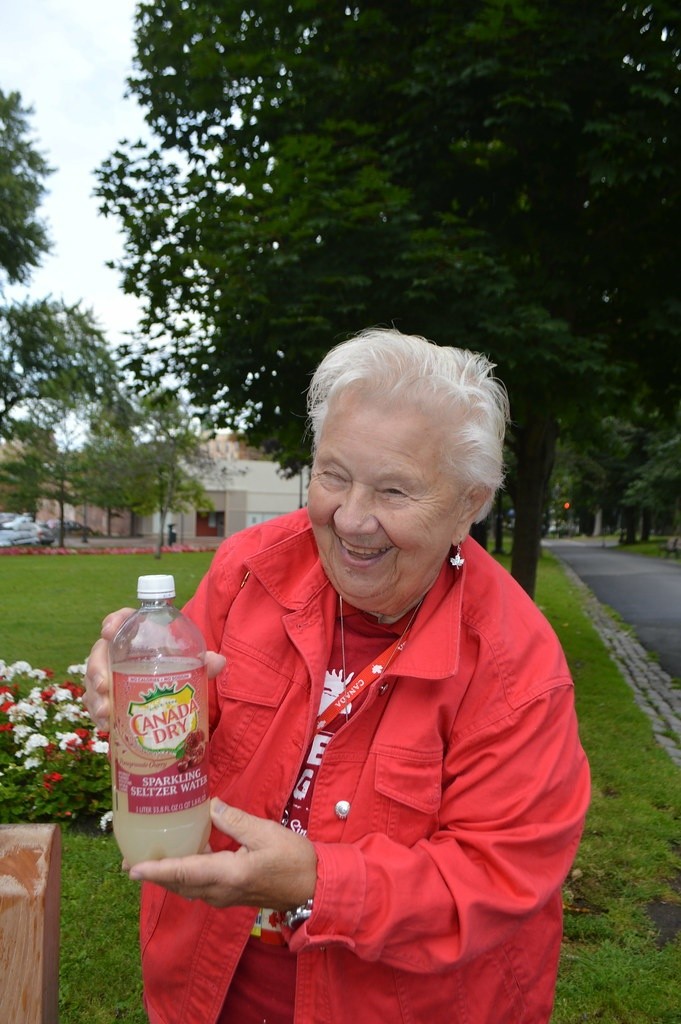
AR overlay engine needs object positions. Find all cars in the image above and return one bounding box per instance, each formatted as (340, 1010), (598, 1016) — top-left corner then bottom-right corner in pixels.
(0, 512), (98, 548)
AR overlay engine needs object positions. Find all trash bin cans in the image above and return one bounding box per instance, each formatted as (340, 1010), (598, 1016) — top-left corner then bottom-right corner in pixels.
(167, 523), (178, 545)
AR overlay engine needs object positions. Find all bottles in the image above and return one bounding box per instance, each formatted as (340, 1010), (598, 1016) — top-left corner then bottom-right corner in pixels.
(108, 575), (212, 867)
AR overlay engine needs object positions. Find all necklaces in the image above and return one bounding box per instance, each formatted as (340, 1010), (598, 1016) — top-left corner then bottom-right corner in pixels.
(340, 595), (423, 722)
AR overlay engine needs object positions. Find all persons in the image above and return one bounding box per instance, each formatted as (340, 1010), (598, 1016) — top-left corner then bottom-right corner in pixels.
(82, 327), (591, 1024)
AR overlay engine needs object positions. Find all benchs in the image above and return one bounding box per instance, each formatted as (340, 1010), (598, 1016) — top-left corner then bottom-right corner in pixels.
(657, 536), (681, 558)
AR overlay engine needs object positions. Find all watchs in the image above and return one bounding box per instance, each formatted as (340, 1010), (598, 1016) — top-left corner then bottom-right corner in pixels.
(284, 899), (313, 931)
